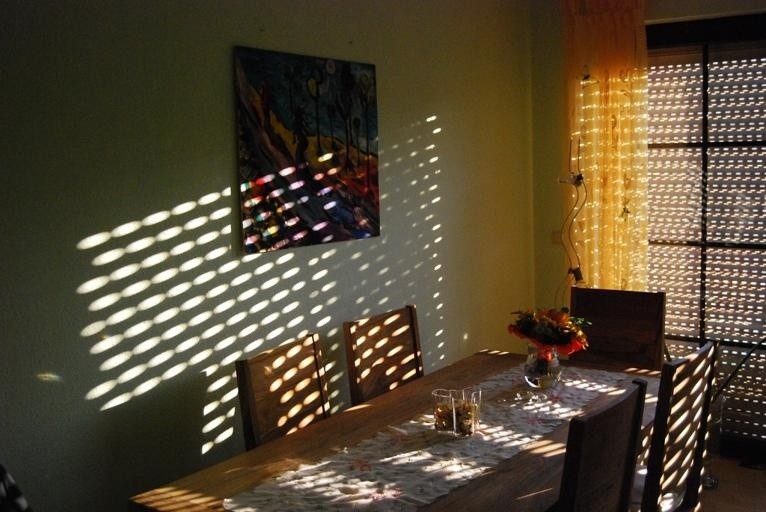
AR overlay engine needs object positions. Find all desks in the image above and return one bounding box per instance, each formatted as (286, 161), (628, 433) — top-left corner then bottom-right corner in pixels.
(127, 349), (661, 511)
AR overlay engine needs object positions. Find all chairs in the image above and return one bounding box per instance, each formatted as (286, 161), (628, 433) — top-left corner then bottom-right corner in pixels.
(569, 287), (667, 373)
(547, 379), (645, 510)
(239, 335), (330, 448)
(635, 339), (715, 510)
(345, 304), (426, 406)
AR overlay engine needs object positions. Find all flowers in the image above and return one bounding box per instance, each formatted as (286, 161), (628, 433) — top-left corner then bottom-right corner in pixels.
(508, 307), (589, 355)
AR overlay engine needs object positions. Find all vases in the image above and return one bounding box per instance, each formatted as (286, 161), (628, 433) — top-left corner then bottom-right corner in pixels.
(523, 347), (562, 388)
